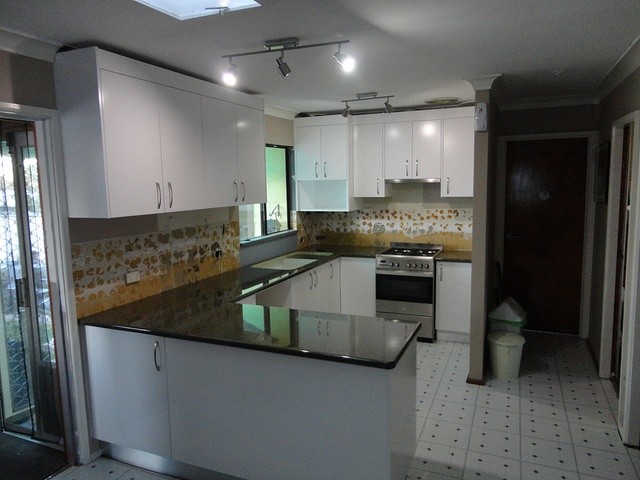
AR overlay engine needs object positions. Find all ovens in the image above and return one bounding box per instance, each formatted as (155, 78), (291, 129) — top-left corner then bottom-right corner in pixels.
(374, 255), (434, 343)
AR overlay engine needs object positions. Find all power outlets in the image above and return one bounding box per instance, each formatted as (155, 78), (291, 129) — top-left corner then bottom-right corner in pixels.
(125, 271), (140, 284)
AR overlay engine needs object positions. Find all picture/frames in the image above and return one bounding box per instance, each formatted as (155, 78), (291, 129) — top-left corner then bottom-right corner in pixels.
(595, 143), (609, 205)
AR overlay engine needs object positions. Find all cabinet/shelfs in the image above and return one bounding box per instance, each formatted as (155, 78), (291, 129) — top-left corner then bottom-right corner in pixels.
(384, 109), (441, 184)
(436, 262), (471, 343)
(293, 114), (362, 212)
(84, 322), (171, 459)
(340, 257), (376, 319)
(203, 81), (267, 210)
(354, 113), (392, 199)
(53, 47), (204, 219)
(441, 106), (474, 198)
(256, 259), (340, 317)
(164, 338), (416, 480)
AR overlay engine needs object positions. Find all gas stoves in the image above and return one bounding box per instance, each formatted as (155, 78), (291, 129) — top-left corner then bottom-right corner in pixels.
(376, 247), (440, 257)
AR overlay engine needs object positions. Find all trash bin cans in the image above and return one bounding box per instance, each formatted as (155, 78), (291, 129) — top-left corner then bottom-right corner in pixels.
(488, 297), (528, 335)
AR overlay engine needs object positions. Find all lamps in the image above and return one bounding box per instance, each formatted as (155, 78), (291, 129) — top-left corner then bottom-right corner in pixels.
(332, 51), (356, 74)
(222, 63), (238, 86)
(383, 102), (393, 113)
(275, 57), (293, 79)
(341, 102), (351, 117)
